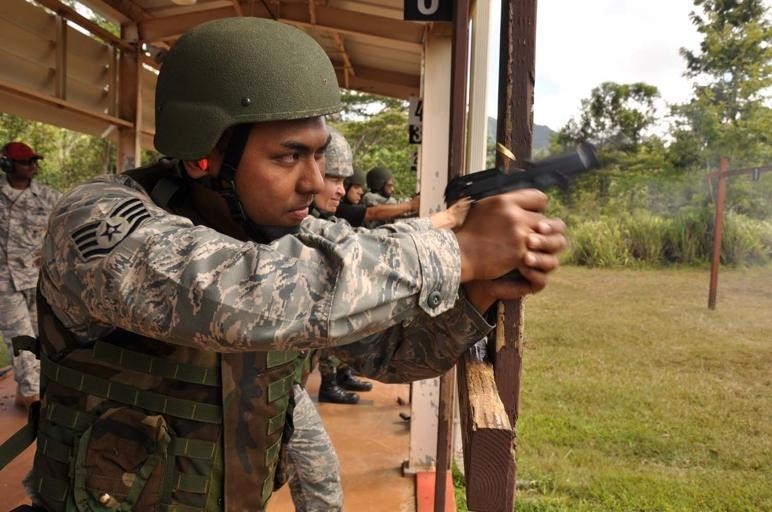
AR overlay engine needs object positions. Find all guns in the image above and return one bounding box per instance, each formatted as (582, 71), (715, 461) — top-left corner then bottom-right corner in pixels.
(447, 141), (596, 281)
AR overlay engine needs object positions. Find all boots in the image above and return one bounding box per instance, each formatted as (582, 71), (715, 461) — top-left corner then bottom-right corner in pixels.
(336, 366), (372, 391)
(318, 373), (360, 403)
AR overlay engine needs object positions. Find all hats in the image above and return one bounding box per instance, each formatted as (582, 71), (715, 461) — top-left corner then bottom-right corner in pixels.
(3, 143), (43, 161)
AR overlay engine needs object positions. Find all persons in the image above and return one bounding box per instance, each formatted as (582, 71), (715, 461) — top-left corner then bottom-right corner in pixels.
(10, 16), (568, 512)
(358, 166), (416, 229)
(0, 142), (64, 410)
(284, 129), (475, 512)
(316, 167), (421, 404)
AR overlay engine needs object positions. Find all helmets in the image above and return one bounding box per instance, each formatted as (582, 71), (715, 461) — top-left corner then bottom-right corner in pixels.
(323, 131), (354, 177)
(154, 17), (344, 161)
(366, 166), (393, 193)
(345, 167), (365, 185)
